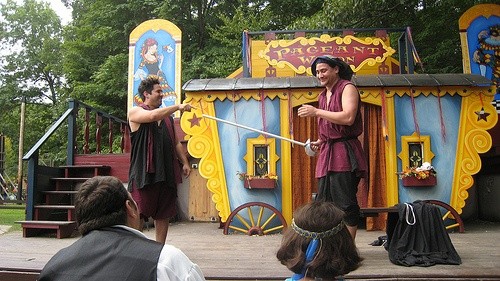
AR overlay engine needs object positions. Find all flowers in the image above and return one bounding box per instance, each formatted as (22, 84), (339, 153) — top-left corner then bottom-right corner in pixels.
(403, 166), (436, 180)
(237, 170), (281, 189)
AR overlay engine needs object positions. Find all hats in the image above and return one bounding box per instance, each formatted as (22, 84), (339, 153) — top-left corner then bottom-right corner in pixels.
(310, 56), (353, 81)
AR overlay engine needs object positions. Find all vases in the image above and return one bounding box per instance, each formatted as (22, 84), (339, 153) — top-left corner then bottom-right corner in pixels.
(244, 179), (277, 189)
(403, 176), (436, 186)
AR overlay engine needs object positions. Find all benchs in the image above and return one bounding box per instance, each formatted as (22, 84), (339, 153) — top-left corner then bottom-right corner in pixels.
(360, 207), (400, 213)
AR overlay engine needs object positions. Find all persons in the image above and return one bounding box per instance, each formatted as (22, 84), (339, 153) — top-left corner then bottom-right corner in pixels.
(37, 175), (205, 281)
(277, 200), (364, 281)
(127, 78), (192, 242)
(298, 55), (368, 241)
